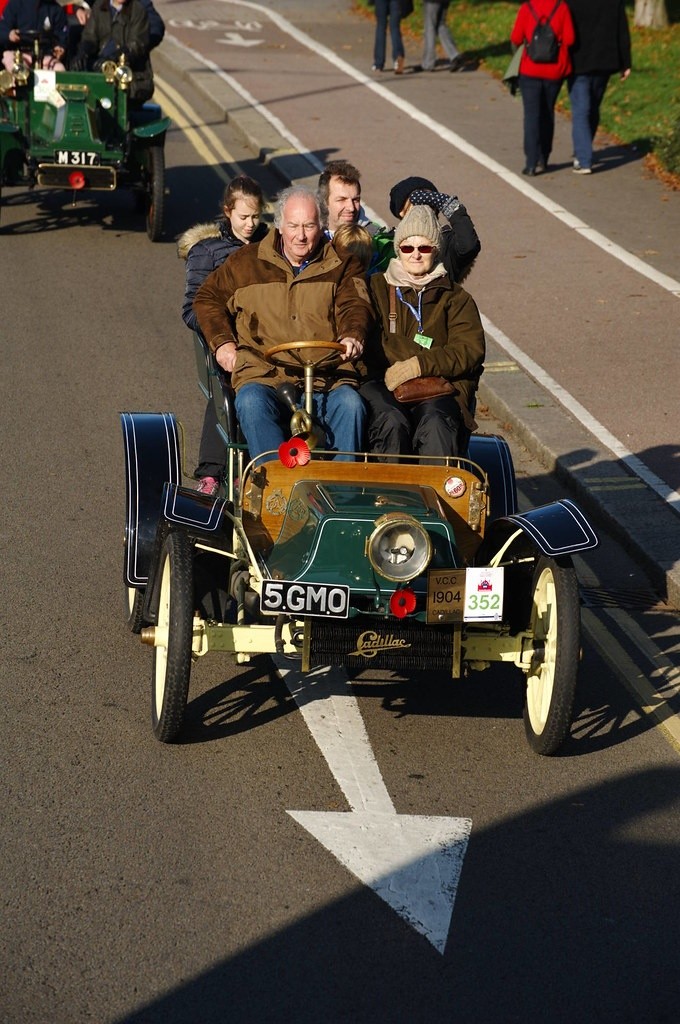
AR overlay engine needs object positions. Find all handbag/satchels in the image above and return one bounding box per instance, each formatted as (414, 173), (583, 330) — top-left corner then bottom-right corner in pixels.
(382, 332), (456, 403)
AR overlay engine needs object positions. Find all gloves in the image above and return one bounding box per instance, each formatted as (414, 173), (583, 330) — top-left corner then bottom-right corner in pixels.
(385, 356), (422, 392)
(410, 188), (458, 214)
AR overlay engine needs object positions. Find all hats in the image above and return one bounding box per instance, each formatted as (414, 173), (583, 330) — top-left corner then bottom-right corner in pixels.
(393, 204), (444, 257)
(390, 177), (441, 220)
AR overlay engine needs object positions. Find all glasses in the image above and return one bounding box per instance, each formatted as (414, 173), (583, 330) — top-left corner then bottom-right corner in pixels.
(398, 245), (437, 254)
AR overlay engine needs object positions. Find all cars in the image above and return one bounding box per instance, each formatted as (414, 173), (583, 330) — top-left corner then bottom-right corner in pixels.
(116, 341), (595, 758)
(1, 29), (172, 244)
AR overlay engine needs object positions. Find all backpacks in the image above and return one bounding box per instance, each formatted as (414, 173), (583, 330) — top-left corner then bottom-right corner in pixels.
(524, 1), (561, 64)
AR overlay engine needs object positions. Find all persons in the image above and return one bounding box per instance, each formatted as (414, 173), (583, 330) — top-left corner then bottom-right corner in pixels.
(332, 224), (374, 261)
(510, 0), (575, 175)
(367, 0), (414, 74)
(319, 163), (383, 235)
(567, 0), (631, 174)
(372, 176), (480, 284)
(359, 204), (484, 466)
(0, 0), (164, 110)
(178, 175), (269, 496)
(194, 188), (372, 503)
(414, 0), (464, 73)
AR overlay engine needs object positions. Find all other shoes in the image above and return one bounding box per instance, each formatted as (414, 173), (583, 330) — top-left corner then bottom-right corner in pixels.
(572, 157), (592, 174)
(538, 160), (547, 169)
(234, 476), (246, 495)
(394, 56), (404, 74)
(522, 167), (536, 176)
(198, 476), (219, 496)
(449, 55), (464, 72)
(371, 65), (383, 73)
(414, 64), (435, 72)
